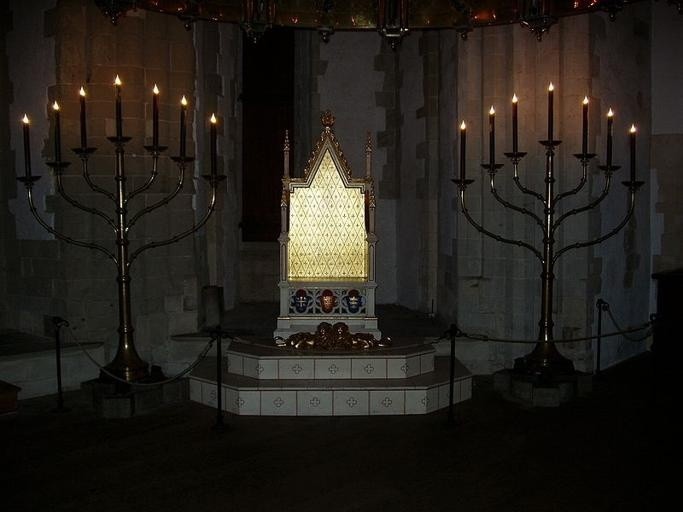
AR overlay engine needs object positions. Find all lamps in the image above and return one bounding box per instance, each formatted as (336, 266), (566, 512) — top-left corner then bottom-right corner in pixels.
(449, 79), (645, 387)
(14, 72), (228, 383)
